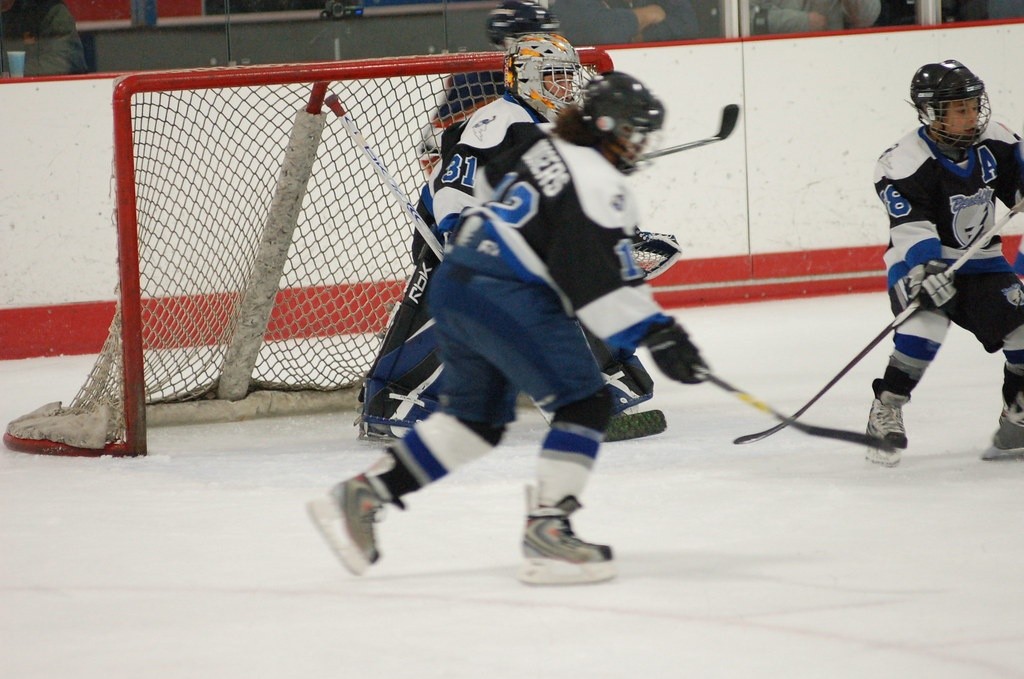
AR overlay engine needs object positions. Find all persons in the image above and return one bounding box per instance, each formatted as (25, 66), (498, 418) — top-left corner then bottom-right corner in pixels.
(865, 60), (1024, 468)
(306, 72), (713, 587)
(353, 0), (710, 447)
(750, 0), (880, 35)
(0, 0), (89, 76)
(869, 0), (962, 26)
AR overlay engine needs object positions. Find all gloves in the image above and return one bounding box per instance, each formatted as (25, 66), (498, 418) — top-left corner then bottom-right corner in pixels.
(906, 260), (957, 311)
(641, 316), (710, 384)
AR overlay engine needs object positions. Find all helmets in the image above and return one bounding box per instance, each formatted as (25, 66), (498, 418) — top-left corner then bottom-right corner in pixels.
(904, 60), (991, 150)
(504, 33), (582, 123)
(582, 70), (665, 176)
(487, 0), (560, 51)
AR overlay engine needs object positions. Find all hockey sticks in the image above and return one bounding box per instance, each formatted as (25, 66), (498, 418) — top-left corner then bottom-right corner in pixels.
(633, 103), (739, 162)
(706, 365), (896, 453)
(734, 199), (1024, 444)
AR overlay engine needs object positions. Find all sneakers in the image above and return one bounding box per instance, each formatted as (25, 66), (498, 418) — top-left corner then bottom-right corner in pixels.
(864, 378), (911, 467)
(980, 391), (1024, 462)
(517, 495), (620, 584)
(305, 472), (405, 580)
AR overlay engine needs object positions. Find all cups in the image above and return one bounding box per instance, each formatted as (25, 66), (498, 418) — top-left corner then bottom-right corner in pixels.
(7, 51), (26, 77)
